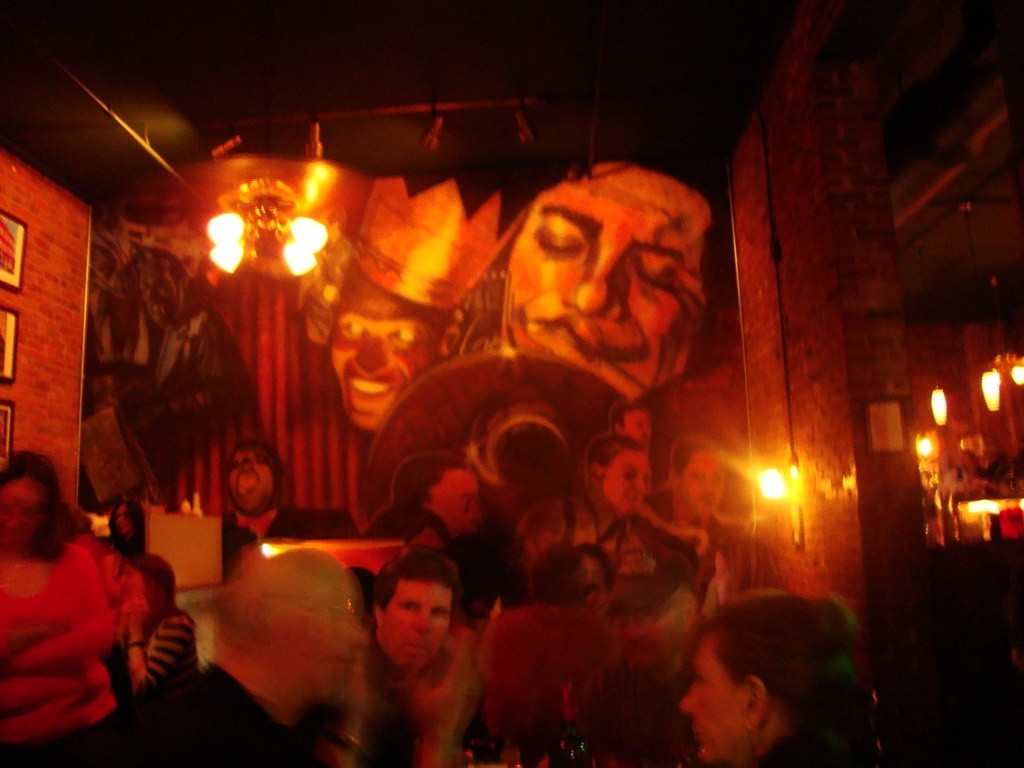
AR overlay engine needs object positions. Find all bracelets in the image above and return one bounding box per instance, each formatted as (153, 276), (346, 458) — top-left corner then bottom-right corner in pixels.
(126, 639), (144, 652)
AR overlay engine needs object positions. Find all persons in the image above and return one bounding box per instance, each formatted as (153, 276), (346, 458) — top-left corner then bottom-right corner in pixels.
(1, 450), (157, 768)
(108, 500), (1024, 768)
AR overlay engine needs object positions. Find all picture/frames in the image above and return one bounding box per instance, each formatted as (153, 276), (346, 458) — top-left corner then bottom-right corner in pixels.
(0, 208), (29, 295)
(0, 304), (20, 385)
(0, 398), (15, 463)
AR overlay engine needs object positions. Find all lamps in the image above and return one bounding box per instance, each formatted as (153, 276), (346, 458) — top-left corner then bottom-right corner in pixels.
(210, 134), (243, 160)
(304, 122), (322, 160)
(514, 109), (538, 146)
(422, 116), (444, 152)
(981, 370), (1001, 412)
(925, 342), (947, 426)
